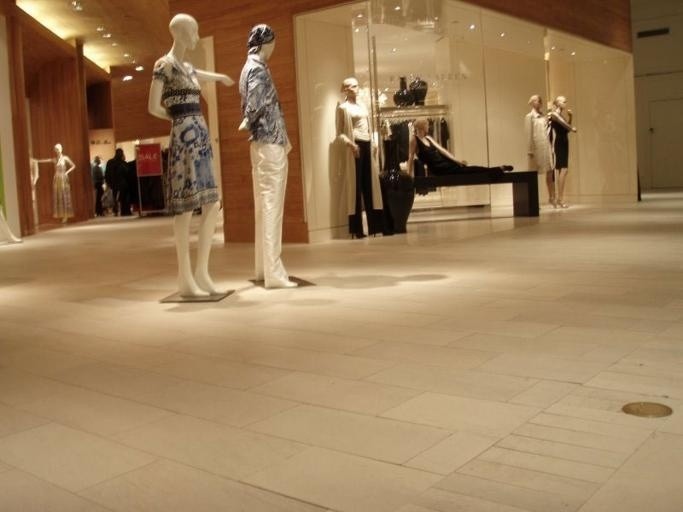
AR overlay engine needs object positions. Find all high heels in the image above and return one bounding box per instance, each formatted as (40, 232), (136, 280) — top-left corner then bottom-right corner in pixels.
(351, 225), (366, 239)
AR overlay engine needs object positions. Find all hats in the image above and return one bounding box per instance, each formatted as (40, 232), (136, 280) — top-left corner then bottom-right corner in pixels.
(244, 23), (275, 53)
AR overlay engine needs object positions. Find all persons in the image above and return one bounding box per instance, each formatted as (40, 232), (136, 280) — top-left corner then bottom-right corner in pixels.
(405, 116), (513, 177)
(523, 94), (556, 208)
(147, 12), (236, 297)
(104, 147), (128, 216)
(29, 155), (39, 229)
(37, 142), (76, 223)
(236, 22), (297, 289)
(91, 156), (105, 217)
(334, 76), (388, 238)
(549, 94), (577, 207)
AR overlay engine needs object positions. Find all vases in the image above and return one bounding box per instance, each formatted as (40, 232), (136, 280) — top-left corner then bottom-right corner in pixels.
(410, 77), (427, 105)
(379, 140), (414, 233)
(393, 77), (415, 106)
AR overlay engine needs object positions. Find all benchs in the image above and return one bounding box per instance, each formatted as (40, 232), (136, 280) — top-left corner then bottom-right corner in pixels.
(414, 171), (539, 217)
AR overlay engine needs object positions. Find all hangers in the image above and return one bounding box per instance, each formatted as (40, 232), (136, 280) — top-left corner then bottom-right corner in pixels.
(374, 112), (413, 126)
